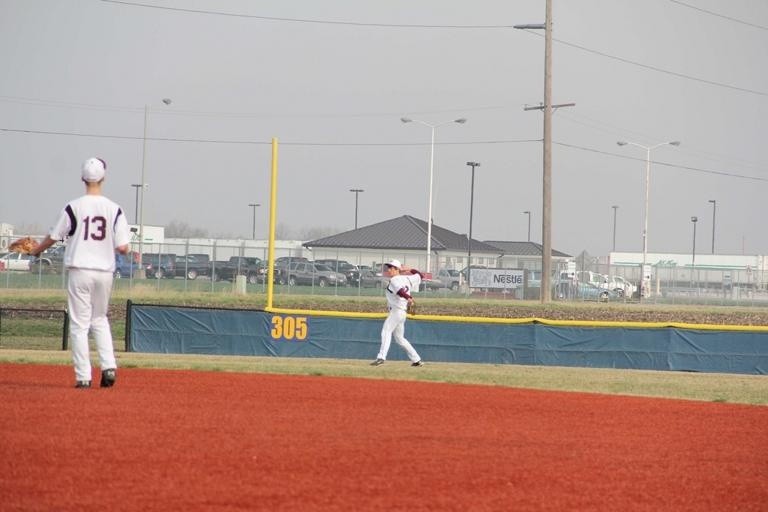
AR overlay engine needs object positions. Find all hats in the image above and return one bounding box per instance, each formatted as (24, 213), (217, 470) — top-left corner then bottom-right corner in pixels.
(383, 257), (403, 270)
(79, 154), (109, 186)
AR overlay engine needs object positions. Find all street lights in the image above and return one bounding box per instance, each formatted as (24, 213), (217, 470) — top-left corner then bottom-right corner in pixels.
(523, 211), (530, 242)
(616, 141), (680, 299)
(248, 204), (260, 239)
(689, 216), (698, 296)
(466, 162), (480, 296)
(349, 189), (363, 230)
(708, 199), (715, 255)
(611, 205), (619, 251)
(401, 116), (466, 291)
(132, 98), (172, 280)
(131, 184), (142, 225)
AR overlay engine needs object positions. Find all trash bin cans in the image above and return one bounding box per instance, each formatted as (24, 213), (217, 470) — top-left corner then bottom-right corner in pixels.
(237, 275), (247, 294)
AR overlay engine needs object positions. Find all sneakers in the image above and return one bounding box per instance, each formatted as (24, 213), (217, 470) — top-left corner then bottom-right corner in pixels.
(369, 357), (386, 369)
(72, 378), (95, 393)
(98, 365), (118, 389)
(410, 358), (426, 370)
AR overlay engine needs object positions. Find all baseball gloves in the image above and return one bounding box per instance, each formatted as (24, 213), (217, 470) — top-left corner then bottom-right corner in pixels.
(9, 237), (40, 255)
(406, 299), (415, 315)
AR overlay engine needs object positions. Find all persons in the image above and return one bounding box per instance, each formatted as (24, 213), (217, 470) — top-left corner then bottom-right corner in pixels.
(9, 157), (133, 388)
(370, 260), (424, 367)
